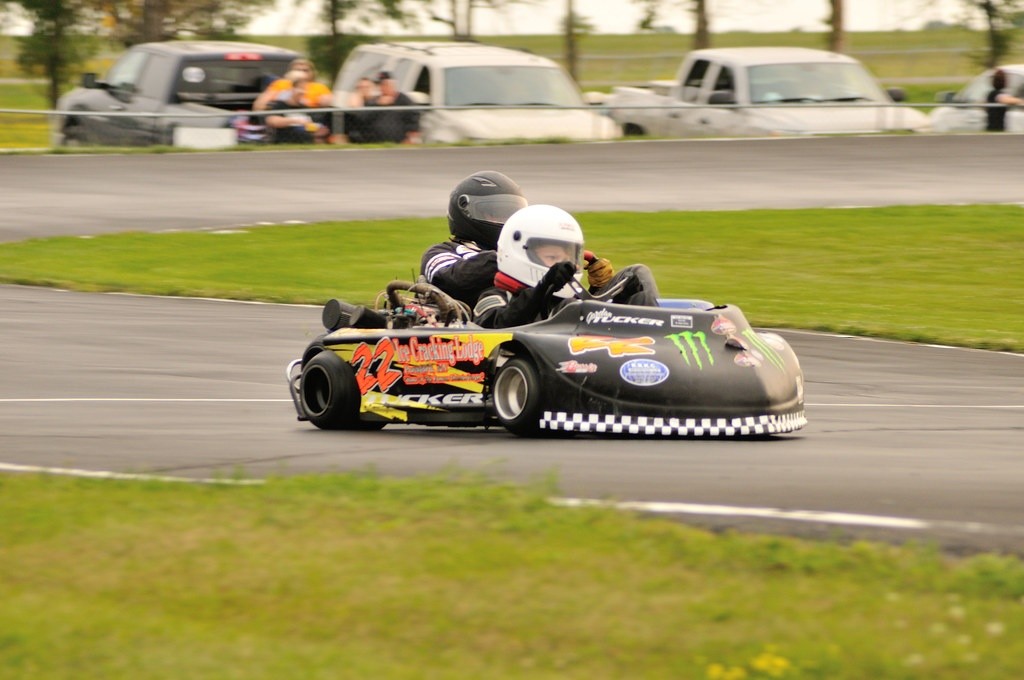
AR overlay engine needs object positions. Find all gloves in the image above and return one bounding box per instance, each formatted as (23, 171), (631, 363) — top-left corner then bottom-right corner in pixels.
(584, 252), (614, 291)
(524, 262), (581, 311)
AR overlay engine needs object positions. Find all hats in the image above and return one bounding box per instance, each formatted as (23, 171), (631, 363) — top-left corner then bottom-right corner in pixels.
(373, 72), (399, 83)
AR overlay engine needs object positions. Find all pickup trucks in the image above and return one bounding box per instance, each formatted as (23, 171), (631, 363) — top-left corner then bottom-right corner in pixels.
(581, 44), (930, 144)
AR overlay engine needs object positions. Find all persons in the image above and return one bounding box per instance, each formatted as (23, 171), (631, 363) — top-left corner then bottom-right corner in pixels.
(473, 205), (657, 330)
(981, 70), (1024, 131)
(251, 59), (422, 146)
(419, 172), (527, 314)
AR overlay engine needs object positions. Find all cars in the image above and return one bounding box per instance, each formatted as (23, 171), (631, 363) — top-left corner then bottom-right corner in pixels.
(332, 38), (627, 146)
(927, 62), (1023, 134)
(52, 40), (311, 151)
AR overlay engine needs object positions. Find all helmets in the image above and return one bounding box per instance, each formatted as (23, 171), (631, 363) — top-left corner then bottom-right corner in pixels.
(493, 205), (583, 298)
(446, 171), (525, 251)
